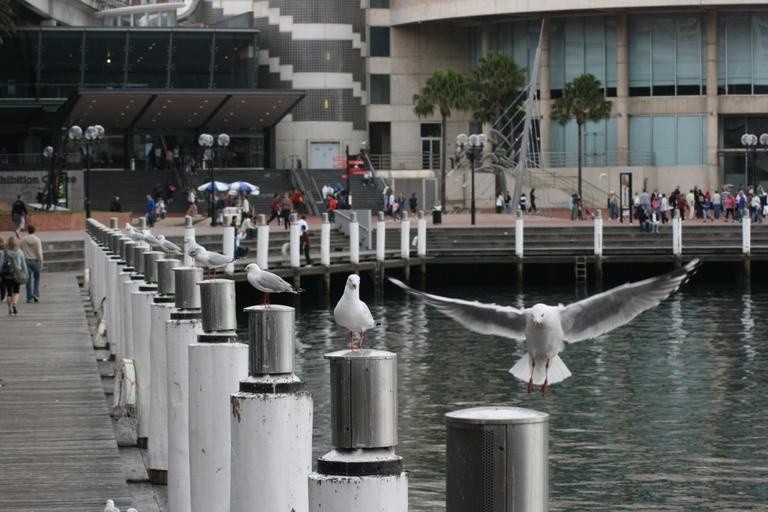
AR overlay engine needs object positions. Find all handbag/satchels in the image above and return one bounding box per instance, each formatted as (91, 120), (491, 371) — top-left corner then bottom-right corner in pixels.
(13, 267), (27, 285)
(0, 257), (11, 279)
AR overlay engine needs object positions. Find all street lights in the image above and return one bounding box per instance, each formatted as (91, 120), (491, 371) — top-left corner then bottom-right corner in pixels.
(42, 146), (55, 212)
(69, 124), (104, 217)
(457, 134), (488, 226)
(740, 132), (768, 194)
(345, 141), (370, 207)
(197, 132), (231, 227)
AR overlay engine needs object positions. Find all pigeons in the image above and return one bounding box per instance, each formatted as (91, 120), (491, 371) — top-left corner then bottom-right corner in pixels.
(127, 227), (145, 246)
(155, 234), (184, 259)
(141, 230), (160, 252)
(183, 238), (206, 267)
(244, 263), (306, 308)
(388, 257), (702, 396)
(104, 499), (120, 512)
(334, 274), (381, 352)
(125, 222), (137, 232)
(191, 246), (234, 280)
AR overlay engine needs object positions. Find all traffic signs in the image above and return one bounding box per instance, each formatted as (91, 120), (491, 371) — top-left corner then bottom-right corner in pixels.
(330, 155), (369, 178)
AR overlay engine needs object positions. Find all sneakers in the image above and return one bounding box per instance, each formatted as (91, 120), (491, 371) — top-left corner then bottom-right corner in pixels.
(33, 296), (40, 304)
(26, 298), (32, 303)
(8, 305), (18, 315)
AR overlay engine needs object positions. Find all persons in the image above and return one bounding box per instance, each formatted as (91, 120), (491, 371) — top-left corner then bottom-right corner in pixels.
(11, 193), (28, 237)
(34, 186), (43, 210)
(0, 237), (6, 302)
(20, 225), (42, 304)
(110, 193), (124, 211)
(142, 133), (419, 270)
(495, 181), (766, 234)
(5, 236), (30, 313)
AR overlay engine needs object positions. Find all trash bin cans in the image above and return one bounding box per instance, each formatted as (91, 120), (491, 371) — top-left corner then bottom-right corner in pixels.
(130, 158), (136, 171)
(297, 159), (302, 168)
(432, 206), (442, 224)
